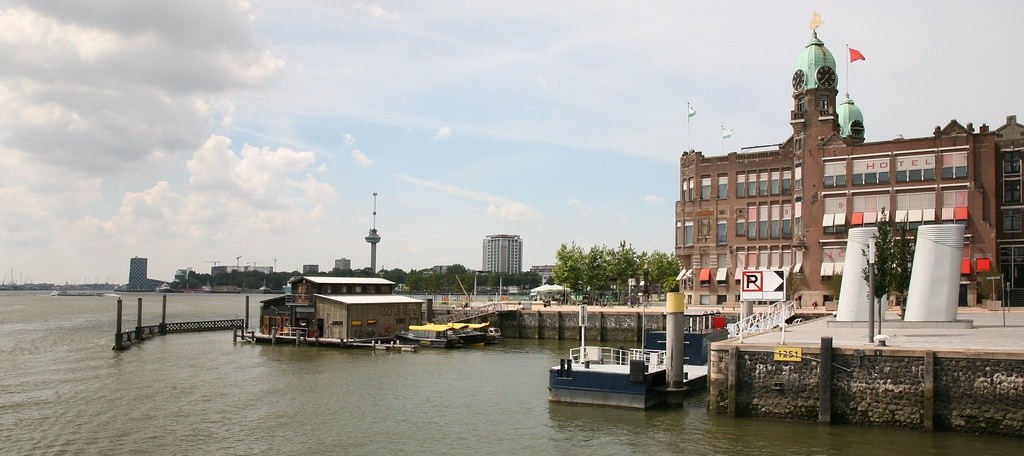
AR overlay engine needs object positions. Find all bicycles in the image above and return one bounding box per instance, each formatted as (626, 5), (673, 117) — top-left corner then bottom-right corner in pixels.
(600, 296), (613, 309)
(628, 298), (651, 309)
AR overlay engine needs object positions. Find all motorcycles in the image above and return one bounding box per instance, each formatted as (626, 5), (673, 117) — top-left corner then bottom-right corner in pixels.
(543, 299), (551, 308)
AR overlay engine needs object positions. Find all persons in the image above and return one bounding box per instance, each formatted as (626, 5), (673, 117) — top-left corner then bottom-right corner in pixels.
(811, 299), (819, 309)
(794, 293), (803, 309)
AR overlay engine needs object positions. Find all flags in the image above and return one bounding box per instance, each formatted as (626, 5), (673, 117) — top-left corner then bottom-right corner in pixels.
(849, 47), (865, 63)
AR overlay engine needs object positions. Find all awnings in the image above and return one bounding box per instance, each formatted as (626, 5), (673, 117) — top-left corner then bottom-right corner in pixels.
(954, 206), (968, 220)
(863, 211), (877, 224)
(877, 211), (889, 222)
(820, 262), (834, 277)
(793, 263), (802, 273)
(834, 213), (846, 226)
(923, 208), (936, 221)
(976, 258), (991, 272)
(676, 268), (686, 281)
(715, 267), (727, 281)
(907, 209), (922, 222)
(781, 266), (790, 280)
(681, 268), (693, 280)
(734, 267), (744, 280)
(960, 259), (972, 274)
(895, 210), (907, 223)
(699, 268), (711, 281)
(822, 214), (834, 227)
(941, 207), (954, 220)
(834, 263), (845, 275)
(850, 211), (863, 225)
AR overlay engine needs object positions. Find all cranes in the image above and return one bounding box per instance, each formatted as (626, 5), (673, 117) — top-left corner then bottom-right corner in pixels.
(206, 261), (221, 267)
(235, 256), (242, 270)
(273, 258), (277, 272)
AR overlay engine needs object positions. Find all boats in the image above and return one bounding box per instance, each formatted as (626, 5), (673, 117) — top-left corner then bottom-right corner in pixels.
(155, 280), (170, 292)
(484, 326), (503, 344)
(258, 279), (271, 294)
(50, 289), (106, 297)
(202, 283), (213, 293)
(394, 322), (490, 349)
(282, 280), (292, 295)
